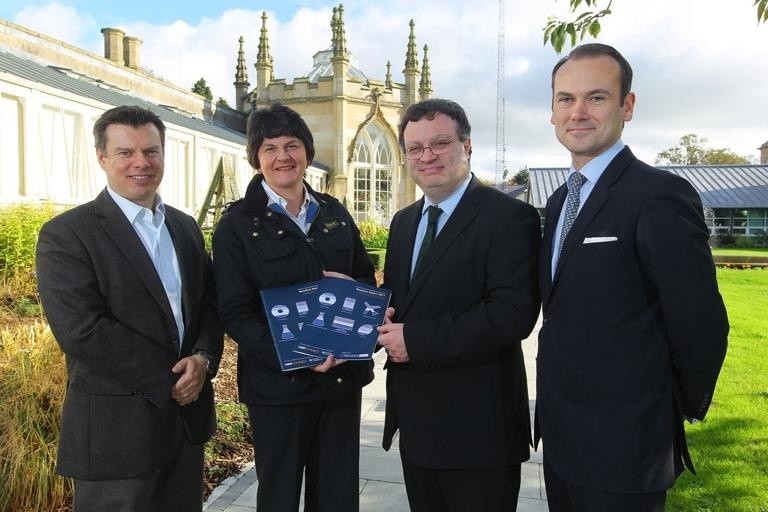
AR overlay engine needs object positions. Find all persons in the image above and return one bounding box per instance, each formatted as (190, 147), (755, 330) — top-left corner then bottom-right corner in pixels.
(35, 105), (224, 512)
(374, 99), (542, 512)
(533, 44), (729, 511)
(212, 102), (377, 512)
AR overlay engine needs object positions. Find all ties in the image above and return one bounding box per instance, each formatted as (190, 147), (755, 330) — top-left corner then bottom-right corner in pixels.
(557, 173), (588, 262)
(411, 207), (443, 282)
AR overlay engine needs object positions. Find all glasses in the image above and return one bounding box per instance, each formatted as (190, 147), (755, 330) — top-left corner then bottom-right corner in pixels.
(405, 139), (459, 160)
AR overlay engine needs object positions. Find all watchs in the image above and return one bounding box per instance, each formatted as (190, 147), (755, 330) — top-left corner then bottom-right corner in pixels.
(196, 350), (217, 376)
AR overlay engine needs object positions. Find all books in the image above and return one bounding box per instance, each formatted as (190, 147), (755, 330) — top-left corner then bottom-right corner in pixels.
(291, 277), (394, 362)
(258, 280), (327, 372)
